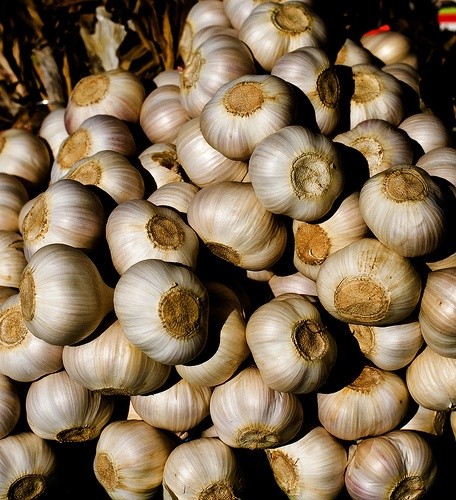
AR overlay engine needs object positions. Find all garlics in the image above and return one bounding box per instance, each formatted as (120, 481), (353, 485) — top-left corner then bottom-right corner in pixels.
(0, 1), (455, 499)
